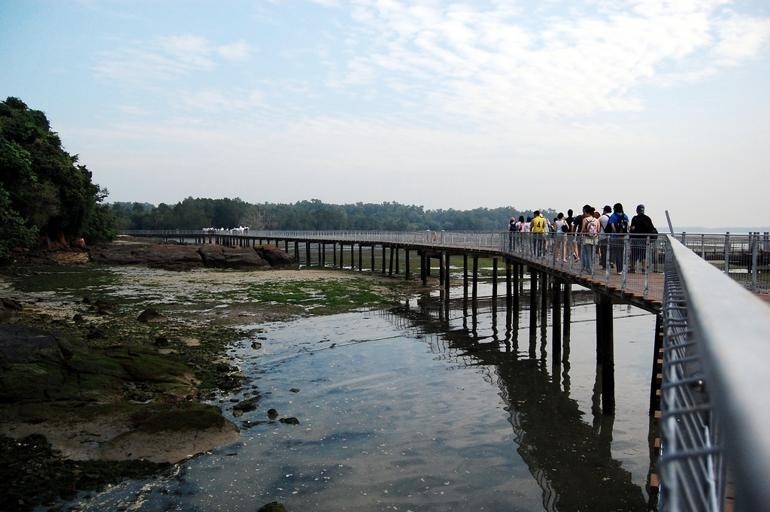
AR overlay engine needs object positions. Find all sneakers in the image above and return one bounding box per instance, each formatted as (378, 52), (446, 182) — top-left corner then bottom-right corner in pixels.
(508, 250), (648, 276)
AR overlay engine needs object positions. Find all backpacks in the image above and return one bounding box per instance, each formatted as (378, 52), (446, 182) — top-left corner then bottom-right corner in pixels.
(585, 217), (599, 238)
(605, 215), (613, 235)
(614, 212), (628, 237)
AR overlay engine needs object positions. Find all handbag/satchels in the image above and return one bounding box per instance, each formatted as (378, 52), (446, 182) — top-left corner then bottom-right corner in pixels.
(561, 225), (568, 233)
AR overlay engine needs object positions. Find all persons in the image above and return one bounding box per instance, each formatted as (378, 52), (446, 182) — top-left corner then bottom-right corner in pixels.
(506, 203), (658, 275)
